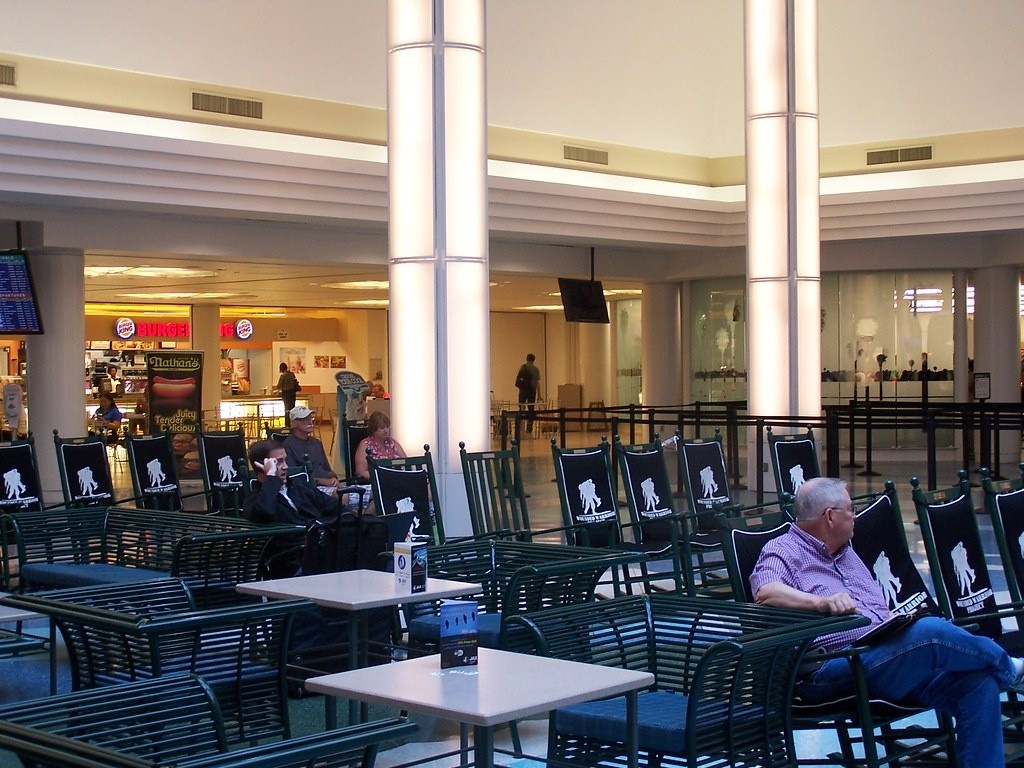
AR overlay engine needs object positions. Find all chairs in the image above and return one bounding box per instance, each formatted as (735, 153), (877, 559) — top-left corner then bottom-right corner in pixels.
(0, 422), (1024, 768)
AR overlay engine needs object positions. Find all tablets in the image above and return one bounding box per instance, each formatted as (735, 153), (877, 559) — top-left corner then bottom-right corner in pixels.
(852, 611), (933, 647)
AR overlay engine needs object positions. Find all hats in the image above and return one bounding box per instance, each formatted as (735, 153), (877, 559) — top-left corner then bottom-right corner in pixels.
(289, 406), (316, 420)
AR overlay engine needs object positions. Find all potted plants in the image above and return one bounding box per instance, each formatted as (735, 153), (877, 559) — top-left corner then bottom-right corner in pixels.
(876, 354), (888, 380)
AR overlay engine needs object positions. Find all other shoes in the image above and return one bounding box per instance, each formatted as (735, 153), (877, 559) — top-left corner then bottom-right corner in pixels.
(1004, 658), (1024, 695)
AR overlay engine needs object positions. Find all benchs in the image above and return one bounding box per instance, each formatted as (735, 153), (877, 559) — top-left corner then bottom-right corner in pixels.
(4, 506), (305, 654)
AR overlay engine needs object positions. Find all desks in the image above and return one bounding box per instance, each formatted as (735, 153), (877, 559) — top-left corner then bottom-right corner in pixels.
(304, 645), (655, 768)
(235, 568), (483, 722)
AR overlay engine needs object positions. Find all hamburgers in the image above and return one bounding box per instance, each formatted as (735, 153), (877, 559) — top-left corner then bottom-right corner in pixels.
(171, 433), (202, 470)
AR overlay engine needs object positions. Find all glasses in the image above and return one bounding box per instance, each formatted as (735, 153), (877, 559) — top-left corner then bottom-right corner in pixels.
(821, 507), (857, 518)
(294, 417), (315, 423)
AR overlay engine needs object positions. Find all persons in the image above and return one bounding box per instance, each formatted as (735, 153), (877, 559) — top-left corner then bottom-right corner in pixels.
(277, 362), (301, 410)
(106, 365), (121, 394)
(365, 381), (385, 398)
(87, 393), (122, 444)
(749, 477), (1024, 768)
(517, 354), (542, 434)
(135, 384), (149, 430)
(355, 412), (408, 515)
(282, 406), (349, 504)
(244, 441), (343, 526)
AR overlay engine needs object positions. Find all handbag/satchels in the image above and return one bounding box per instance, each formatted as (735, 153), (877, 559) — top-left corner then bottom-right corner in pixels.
(291, 372), (301, 391)
(514, 364), (531, 392)
(345, 393), (366, 420)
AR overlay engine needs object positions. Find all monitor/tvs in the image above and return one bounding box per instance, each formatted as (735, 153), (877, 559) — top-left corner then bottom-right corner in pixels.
(558, 277), (609, 323)
(0, 250), (44, 335)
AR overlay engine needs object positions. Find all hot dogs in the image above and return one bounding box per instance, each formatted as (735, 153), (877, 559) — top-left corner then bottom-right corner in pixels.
(152, 376), (196, 397)
